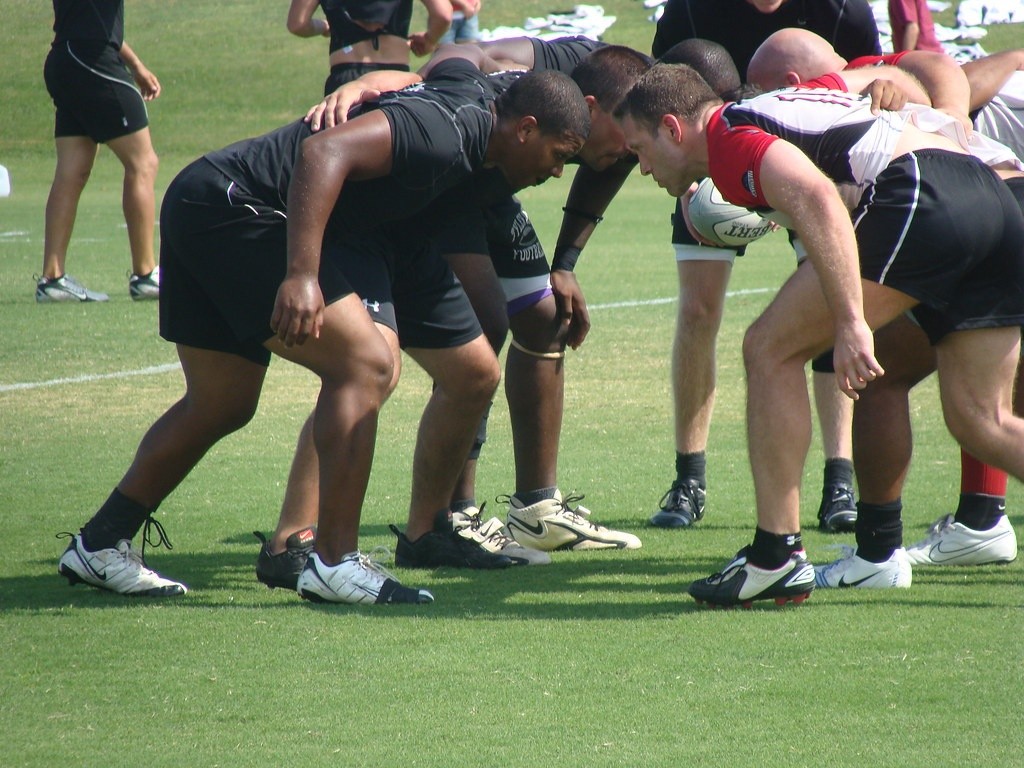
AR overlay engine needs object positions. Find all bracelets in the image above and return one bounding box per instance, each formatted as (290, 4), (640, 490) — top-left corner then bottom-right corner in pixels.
(552, 245), (583, 270)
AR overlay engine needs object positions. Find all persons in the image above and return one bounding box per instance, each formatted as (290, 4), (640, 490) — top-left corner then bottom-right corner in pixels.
(612, 64), (1023, 608)
(288, 0), (452, 91)
(256, 34), (743, 589)
(441, 0), (481, 45)
(888, 0), (945, 53)
(644, 0), (884, 528)
(57, 39), (590, 606)
(745, 28), (1023, 592)
(36, 0), (161, 301)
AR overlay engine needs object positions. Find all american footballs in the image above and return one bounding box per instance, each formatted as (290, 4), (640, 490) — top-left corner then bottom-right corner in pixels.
(688, 177), (774, 246)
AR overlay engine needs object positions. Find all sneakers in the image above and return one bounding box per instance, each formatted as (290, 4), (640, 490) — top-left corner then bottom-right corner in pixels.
(817, 482), (858, 531)
(253, 525), (317, 591)
(297, 546), (435, 604)
(452, 501), (551, 564)
(55, 531), (189, 596)
(494, 488), (642, 552)
(689, 544), (816, 611)
(651, 479), (707, 528)
(906, 513), (1018, 566)
(126, 265), (160, 300)
(32, 273), (110, 302)
(389, 507), (512, 568)
(813, 545), (912, 590)
